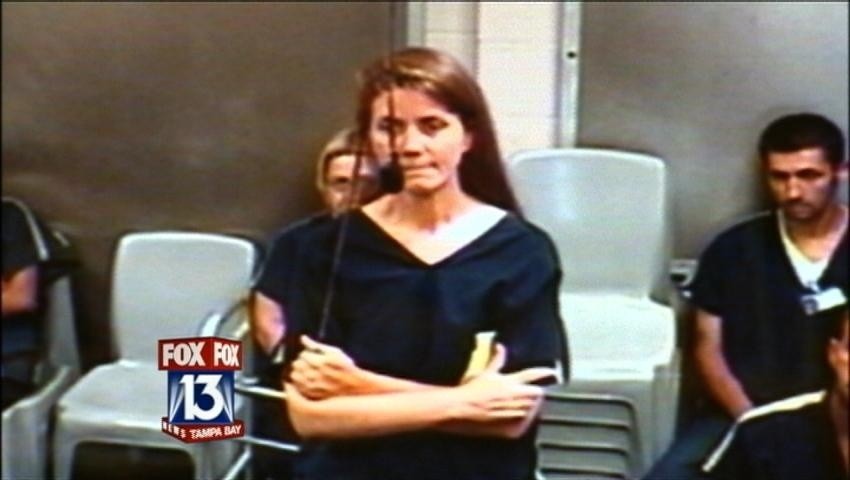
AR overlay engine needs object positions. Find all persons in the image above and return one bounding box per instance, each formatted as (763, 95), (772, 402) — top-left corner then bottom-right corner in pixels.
(0, 194), (82, 417)
(643, 112), (850, 479)
(694, 314), (850, 480)
(250, 127), (392, 480)
(281, 46), (571, 480)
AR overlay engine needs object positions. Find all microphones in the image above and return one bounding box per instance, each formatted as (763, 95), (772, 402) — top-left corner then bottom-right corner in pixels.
(375, 75), (405, 193)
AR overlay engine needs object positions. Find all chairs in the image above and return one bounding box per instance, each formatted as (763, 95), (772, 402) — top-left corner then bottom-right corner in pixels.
(54, 231), (262, 480)
(505, 146), (682, 479)
(2, 275), (82, 480)
